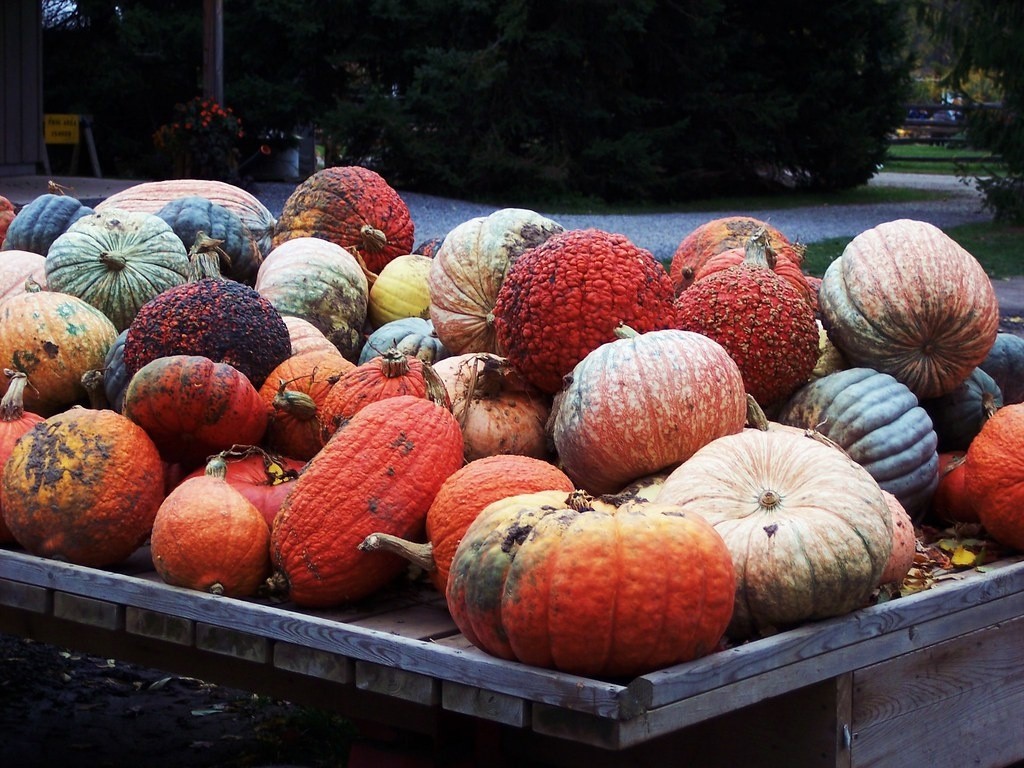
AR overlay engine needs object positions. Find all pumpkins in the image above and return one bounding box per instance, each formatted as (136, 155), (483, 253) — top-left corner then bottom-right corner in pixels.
(0, 165), (1024, 674)
